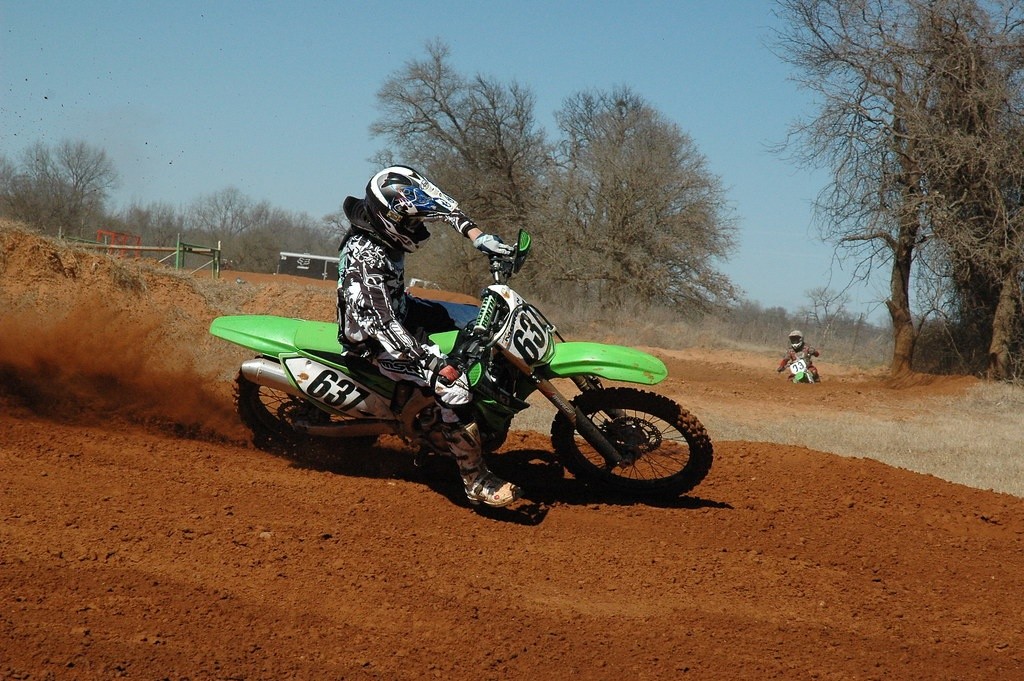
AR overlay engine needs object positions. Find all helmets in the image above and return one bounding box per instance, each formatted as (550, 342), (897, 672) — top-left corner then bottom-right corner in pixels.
(365, 167), (458, 255)
(787, 329), (804, 350)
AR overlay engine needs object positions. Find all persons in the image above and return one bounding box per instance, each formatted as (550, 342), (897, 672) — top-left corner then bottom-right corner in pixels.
(335, 166), (522, 507)
(777, 330), (822, 384)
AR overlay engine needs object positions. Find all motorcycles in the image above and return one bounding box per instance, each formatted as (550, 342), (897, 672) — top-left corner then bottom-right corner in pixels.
(209, 229), (713, 499)
(779, 353), (815, 384)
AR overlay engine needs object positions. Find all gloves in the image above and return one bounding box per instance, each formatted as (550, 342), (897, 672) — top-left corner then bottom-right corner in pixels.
(423, 354), (460, 384)
(808, 346), (816, 354)
(777, 367), (783, 372)
(473, 233), (515, 259)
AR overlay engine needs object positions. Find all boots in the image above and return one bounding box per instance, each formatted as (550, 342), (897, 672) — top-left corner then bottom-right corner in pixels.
(438, 422), (523, 509)
